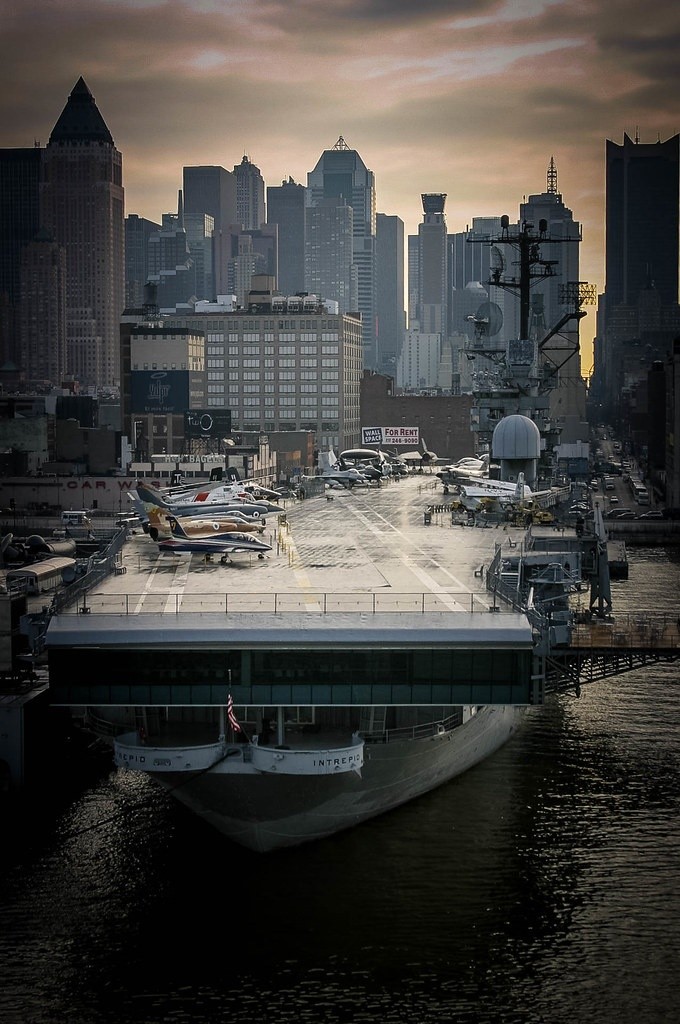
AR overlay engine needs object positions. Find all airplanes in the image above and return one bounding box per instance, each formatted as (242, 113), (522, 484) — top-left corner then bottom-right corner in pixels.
(126, 467), (284, 562)
(302, 437), (550, 517)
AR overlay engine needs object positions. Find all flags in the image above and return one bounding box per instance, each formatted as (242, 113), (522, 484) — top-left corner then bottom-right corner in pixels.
(227, 688), (239, 732)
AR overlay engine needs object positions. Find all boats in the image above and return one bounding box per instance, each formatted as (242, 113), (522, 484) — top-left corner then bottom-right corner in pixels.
(109, 668), (529, 857)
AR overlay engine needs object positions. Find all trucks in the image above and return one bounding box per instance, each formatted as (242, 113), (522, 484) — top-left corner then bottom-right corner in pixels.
(61, 510), (91, 526)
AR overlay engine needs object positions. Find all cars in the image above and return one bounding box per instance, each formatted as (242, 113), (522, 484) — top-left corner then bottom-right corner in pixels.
(568, 433), (662, 518)
